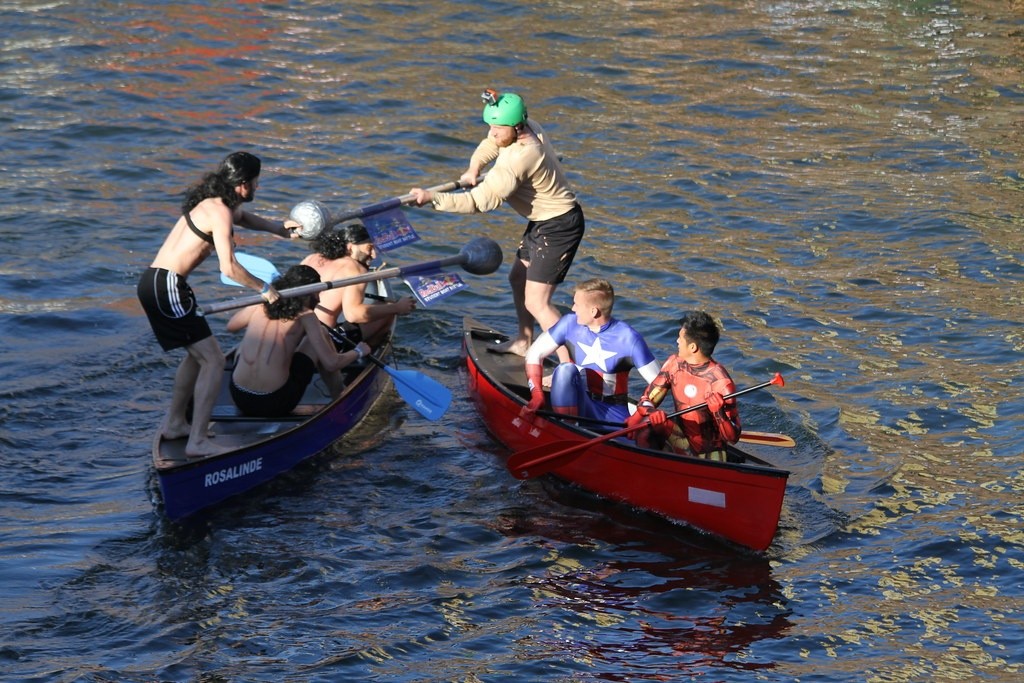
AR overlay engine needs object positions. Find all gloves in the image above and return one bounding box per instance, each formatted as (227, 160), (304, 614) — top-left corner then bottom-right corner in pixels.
(624, 412), (644, 440)
(523, 392), (545, 415)
(705, 392), (724, 413)
(650, 410), (668, 427)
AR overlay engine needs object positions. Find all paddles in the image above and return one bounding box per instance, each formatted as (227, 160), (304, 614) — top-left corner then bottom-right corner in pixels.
(505, 372), (785, 482)
(318, 321), (455, 422)
(218, 250), (413, 305)
(533, 409), (797, 448)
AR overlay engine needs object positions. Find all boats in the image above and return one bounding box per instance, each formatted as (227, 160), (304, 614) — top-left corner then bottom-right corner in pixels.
(462, 316), (791, 553)
(149, 266), (398, 524)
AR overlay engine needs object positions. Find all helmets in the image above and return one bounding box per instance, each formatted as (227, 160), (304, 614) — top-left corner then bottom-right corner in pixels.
(483, 92), (528, 126)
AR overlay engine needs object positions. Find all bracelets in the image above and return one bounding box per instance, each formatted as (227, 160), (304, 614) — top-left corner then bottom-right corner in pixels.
(257, 282), (270, 295)
(354, 348), (363, 359)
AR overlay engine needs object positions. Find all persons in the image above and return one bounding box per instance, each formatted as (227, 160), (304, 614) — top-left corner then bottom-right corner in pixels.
(137, 150), (302, 458)
(406, 89), (585, 388)
(623, 310), (741, 463)
(225, 264), (383, 415)
(524, 278), (662, 446)
(300, 225), (416, 355)
